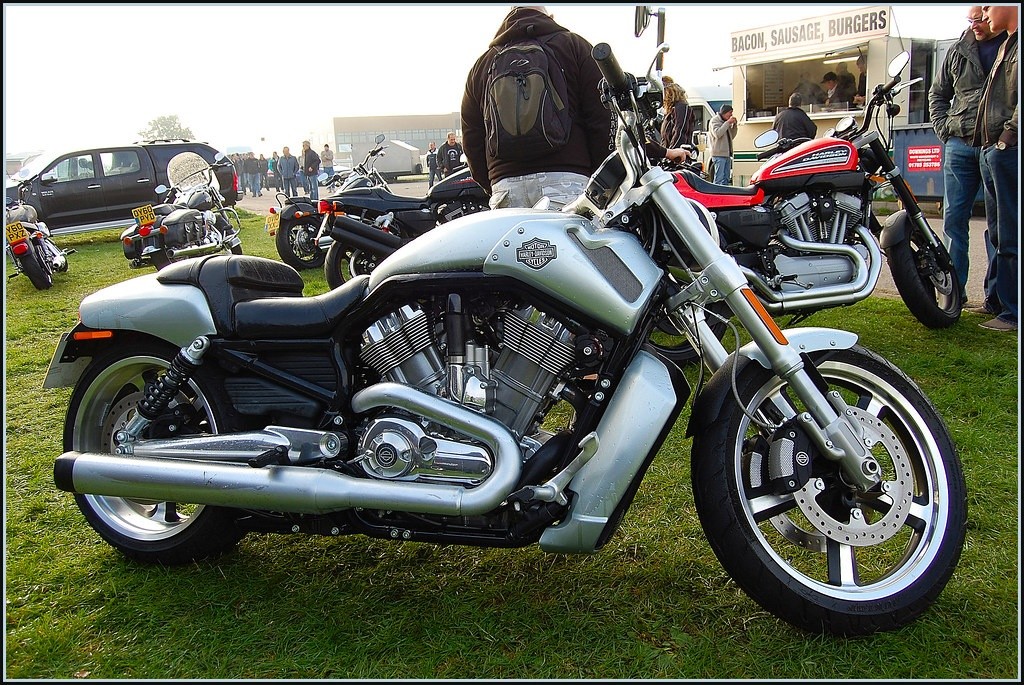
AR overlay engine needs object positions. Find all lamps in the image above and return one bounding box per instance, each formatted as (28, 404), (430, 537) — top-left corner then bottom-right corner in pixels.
(822, 55), (860, 64)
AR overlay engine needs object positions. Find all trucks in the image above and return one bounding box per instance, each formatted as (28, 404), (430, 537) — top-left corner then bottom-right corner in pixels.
(711, 3), (984, 205)
(684, 96), (733, 165)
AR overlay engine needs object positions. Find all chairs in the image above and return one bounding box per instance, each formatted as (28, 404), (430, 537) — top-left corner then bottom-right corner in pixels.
(77, 158), (88, 177)
(87, 161), (94, 178)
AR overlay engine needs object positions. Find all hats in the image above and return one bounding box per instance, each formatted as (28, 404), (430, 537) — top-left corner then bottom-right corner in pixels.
(719, 104), (733, 114)
(820, 71), (839, 84)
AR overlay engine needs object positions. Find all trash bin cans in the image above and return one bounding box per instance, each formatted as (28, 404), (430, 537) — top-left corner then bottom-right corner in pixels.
(893, 122), (944, 199)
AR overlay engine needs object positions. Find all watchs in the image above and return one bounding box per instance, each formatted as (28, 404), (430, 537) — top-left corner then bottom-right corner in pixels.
(998, 142), (1006, 150)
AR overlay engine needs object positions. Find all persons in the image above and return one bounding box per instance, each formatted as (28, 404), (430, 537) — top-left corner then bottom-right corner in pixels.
(853, 55), (867, 106)
(321, 144), (337, 193)
(772, 94), (817, 140)
(708, 104), (737, 185)
(436, 132), (464, 178)
(268, 152), (284, 193)
(277, 147), (299, 199)
(644, 137), (691, 163)
(820, 62), (857, 111)
(970, 5), (1018, 332)
(460, 6), (618, 211)
(258, 154), (270, 191)
(298, 149), (310, 195)
(792, 72), (825, 111)
(303, 141), (321, 199)
(425, 141), (443, 188)
(928, 5), (1008, 308)
(228, 152), (263, 198)
(661, 75), (694, 149)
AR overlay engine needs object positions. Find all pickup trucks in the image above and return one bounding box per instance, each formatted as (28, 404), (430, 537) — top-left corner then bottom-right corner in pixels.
(6, 138), (245, 237)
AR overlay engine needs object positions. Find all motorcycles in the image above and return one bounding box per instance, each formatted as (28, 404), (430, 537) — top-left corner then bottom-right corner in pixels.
(645, 52), (963, 367)
(315, 167), (489, 292)
(5, 174), (77, 292)
(39, 2), (971, 642)
(264, 133), (396, 274)
(118, 150), (244, 271)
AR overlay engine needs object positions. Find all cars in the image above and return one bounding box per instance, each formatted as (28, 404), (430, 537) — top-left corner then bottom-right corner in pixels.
(294, 155), (352, 187)
(267, 169), (275, 188)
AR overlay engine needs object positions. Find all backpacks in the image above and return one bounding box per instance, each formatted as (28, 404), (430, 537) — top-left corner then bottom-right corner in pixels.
(482, 31), (590, 159)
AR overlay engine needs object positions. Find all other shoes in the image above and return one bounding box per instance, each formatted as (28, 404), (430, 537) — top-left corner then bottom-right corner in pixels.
(964, 305), (995, 313)
(978, 311), (1017, 331)
